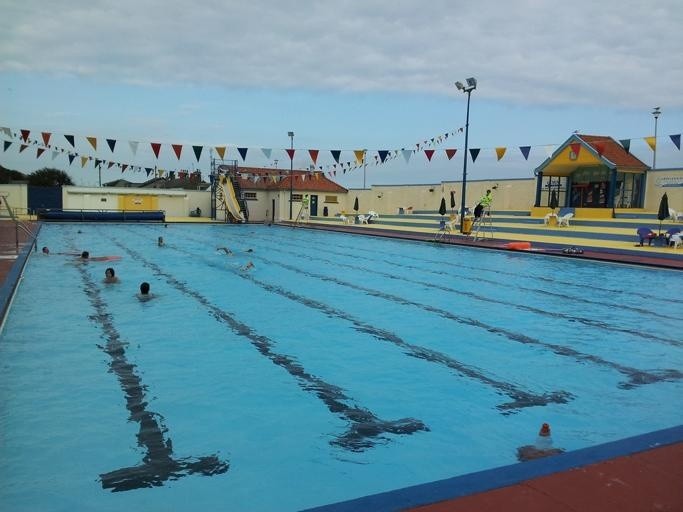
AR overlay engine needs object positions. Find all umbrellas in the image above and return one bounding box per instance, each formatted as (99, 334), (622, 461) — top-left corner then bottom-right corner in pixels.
(449, 190), (455, 214)
(549, 189), (556, 216)
(353, 196), (358, 216)
(438, 197), (446, 221)
(657, 191), (669, 235)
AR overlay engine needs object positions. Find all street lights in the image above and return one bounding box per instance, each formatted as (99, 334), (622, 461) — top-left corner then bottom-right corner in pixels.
(274, 160), (279, 168)
(454, 77), (477, 233)
(362, 149), (368, 188)
(652, 105), (661, 169)
(288, 131), (294, 219)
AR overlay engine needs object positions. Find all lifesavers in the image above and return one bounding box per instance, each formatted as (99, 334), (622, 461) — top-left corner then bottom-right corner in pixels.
(506, 242), (530, 250)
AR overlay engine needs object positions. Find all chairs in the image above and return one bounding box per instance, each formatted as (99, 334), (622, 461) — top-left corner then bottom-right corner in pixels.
(340, 215), (372, 225)
(544, 213), (573, 227)
(434, 206), (493, 242)
(637, 228), (683, 249)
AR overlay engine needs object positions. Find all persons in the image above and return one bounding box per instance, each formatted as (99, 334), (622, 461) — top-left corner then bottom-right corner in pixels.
(134, 281), (154, 301)
(103, 267), (118, 283)
(473, 190), (492, 221)
(217, 246), (232, 254)
(79, 251), (88, 259)
(244, 248), (253, 253)
(244, 261), (256, 271)
(157, 235), (163, 245)
(302, 193), (309, 204)
(41, 246), (49, 254)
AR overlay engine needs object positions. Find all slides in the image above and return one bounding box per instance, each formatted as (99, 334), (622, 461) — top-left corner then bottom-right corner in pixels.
(218, 173), (244, 223)
